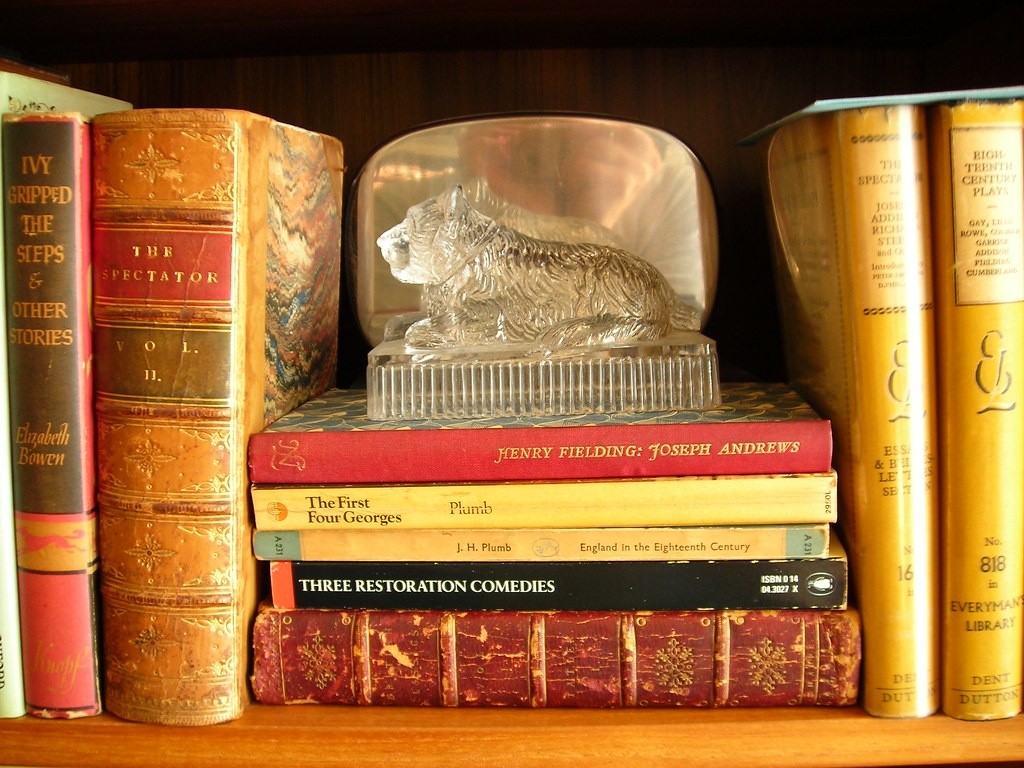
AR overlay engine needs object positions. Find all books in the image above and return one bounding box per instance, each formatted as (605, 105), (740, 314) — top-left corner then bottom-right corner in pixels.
(247, 385), (862, 706)
(0, 73), (132, 719)
(767, 105), (939, 717)
(82, 110), (346, 722)
(935, 99), (1024, 721)
(1, 115), (98, 719)
